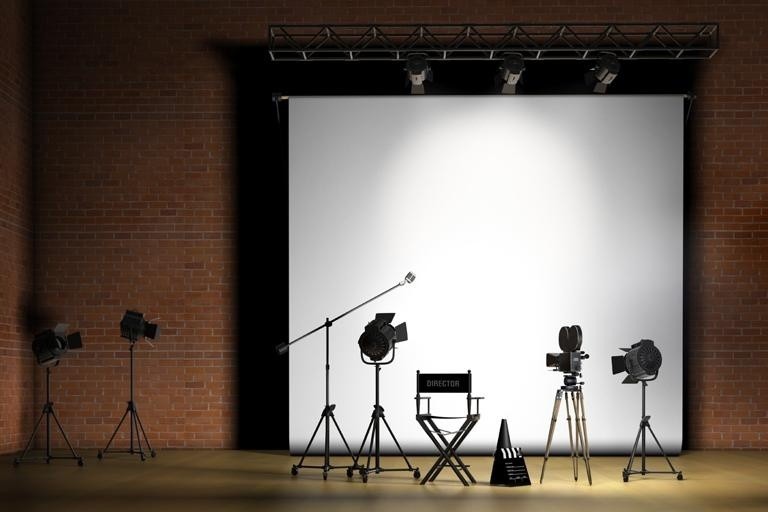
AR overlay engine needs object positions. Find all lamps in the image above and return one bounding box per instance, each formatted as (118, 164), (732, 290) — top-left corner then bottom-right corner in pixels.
(496, 51), (526, 86)
(403, 53), (432, 85)
(590, 52), (621, 84)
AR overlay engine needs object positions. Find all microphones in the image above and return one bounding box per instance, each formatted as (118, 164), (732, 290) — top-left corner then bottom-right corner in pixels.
(406, 270), (416, 285)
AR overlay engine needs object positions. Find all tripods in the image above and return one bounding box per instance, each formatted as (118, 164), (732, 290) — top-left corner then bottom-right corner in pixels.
(14, 373), (84, 466)
(539, 386), (593, 486)
(622, 386), (683, 483)
(290, 326), (366, 481)
(346, 365), (420, 484)
(96, 351), (157, 461)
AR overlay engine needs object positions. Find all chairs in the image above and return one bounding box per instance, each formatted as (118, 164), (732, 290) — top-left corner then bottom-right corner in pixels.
(414, 370), (485, 486)
